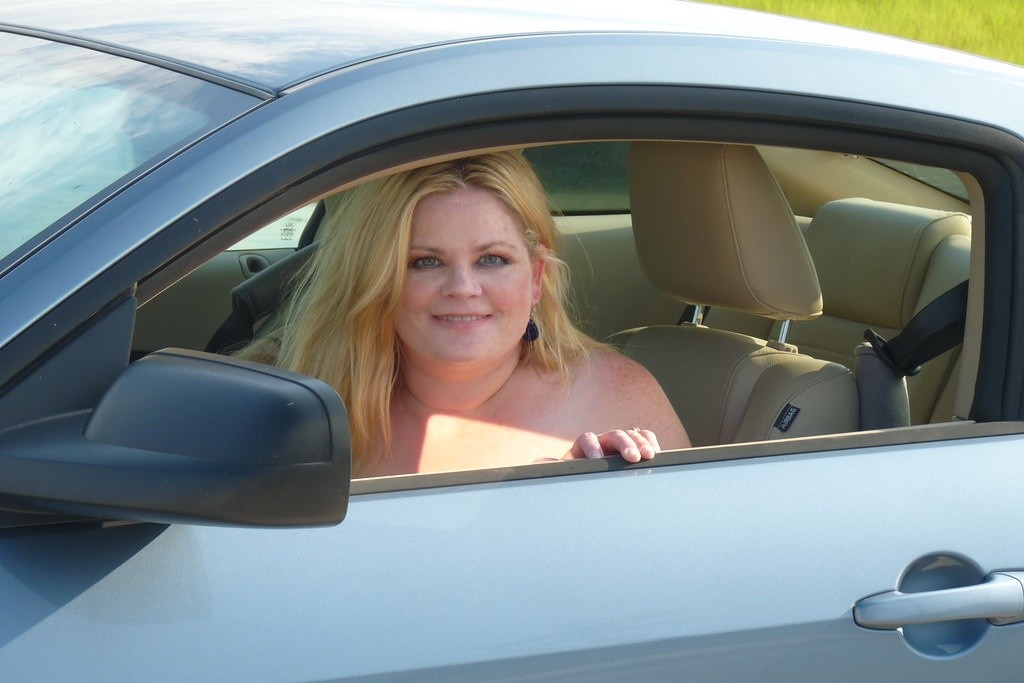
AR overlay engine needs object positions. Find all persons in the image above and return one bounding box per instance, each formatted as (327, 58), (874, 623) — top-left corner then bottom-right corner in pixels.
(233, 152), (693, 479)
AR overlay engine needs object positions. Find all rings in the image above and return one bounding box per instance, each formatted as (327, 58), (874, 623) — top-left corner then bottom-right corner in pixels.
(631, 426), (640, 432)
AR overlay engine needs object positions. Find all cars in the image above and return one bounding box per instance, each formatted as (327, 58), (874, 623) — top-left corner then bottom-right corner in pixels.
(0, 0), (1024, 683)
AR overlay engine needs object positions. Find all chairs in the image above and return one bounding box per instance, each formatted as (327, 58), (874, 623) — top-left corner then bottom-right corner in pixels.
(766, 195), (968, 429)
(602, 140), (858, 448)
